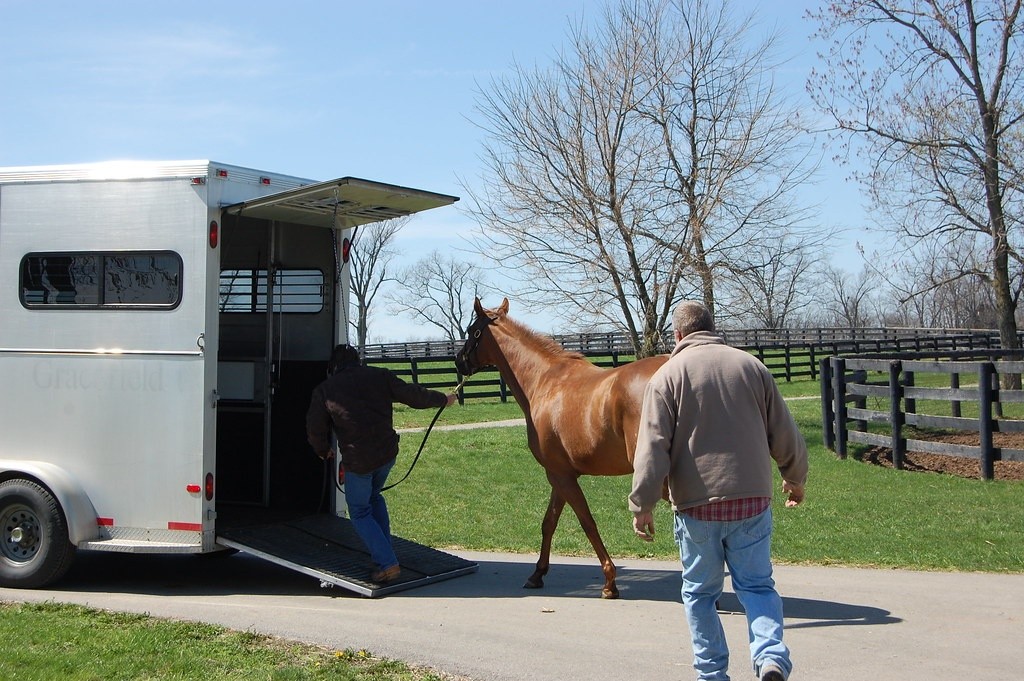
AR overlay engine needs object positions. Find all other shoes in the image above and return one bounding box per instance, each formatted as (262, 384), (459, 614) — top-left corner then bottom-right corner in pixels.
(760, 665), (785, 681)
(370, 563), (401, 581)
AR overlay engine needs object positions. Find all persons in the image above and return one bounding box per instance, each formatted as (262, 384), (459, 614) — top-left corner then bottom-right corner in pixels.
(305, 343), (456, 581)
(628, 300), (809, 681)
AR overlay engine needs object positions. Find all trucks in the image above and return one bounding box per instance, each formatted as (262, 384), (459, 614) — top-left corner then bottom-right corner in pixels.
(1, 159), (482, 602)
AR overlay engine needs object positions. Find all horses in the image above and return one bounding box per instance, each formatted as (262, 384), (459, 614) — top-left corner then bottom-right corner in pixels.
(454, 296), (672, 600)
